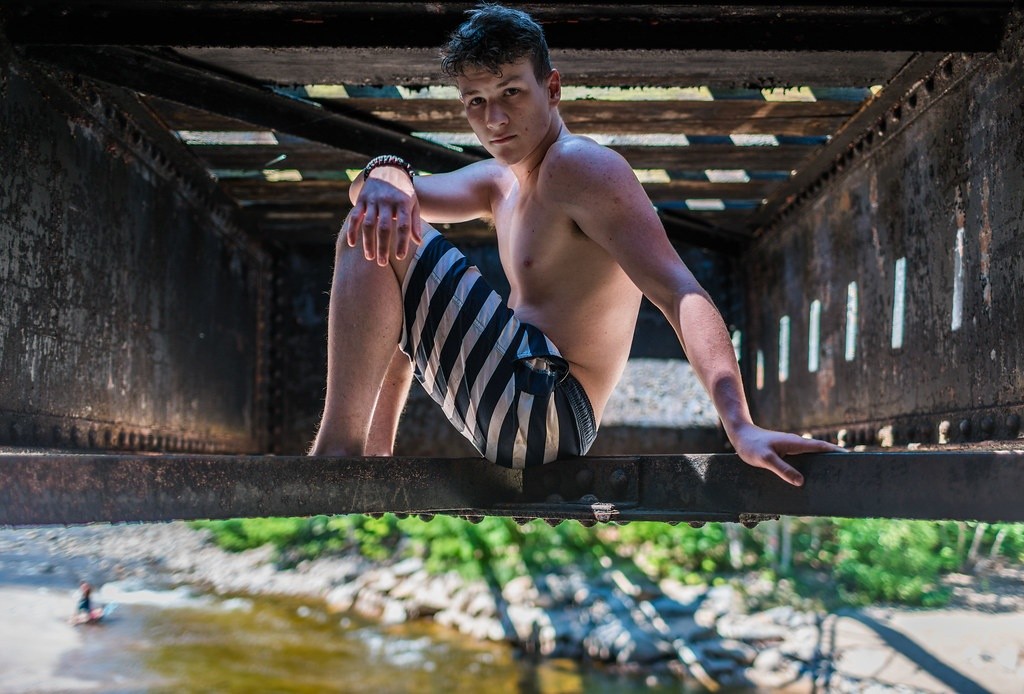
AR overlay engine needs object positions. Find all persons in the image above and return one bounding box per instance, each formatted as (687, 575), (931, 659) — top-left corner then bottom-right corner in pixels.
(309, 0), (849, 487)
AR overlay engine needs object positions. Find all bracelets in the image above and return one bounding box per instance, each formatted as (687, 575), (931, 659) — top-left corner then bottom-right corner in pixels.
(363, 155), (414, 186)
